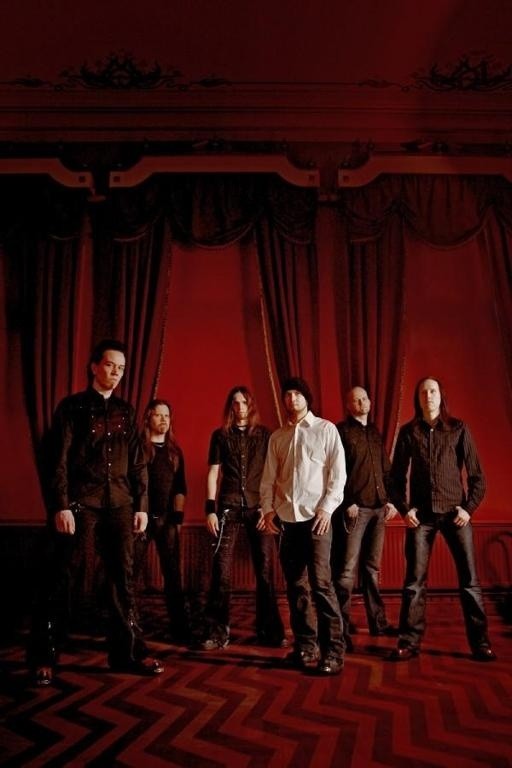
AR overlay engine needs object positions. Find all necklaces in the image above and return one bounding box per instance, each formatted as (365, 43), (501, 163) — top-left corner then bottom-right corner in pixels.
(151, 441), (166, 448)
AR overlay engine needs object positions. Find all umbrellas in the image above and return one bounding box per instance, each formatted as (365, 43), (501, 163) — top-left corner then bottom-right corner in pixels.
(107, 651), (165, 674)
(29, 659), (55, 688)
(391, 640), (424, 660)
(472, 644), (496, 661)
(165, 618), (400, 676)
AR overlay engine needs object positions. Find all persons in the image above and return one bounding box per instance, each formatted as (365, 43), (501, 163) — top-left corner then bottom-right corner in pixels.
(388, 378), (494, 662)
(201, 385), (289, 649)
(28, 342), (165, 684)
(315, 385), (391, 652)
(259, 378), (347, 672)
(127, 400), (194, 647)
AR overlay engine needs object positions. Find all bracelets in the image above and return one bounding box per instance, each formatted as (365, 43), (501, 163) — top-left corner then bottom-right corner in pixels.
(259, 505), (261, 508)
(173, 511), (184, 528)
(205, 499), (216, 515)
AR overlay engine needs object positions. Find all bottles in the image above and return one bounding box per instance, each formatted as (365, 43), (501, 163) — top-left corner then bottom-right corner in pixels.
(282, 377), (313, 407)
